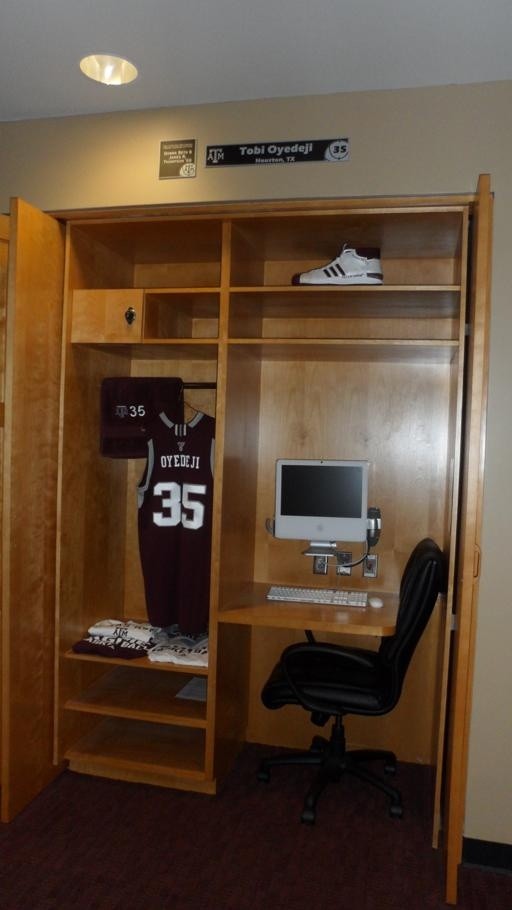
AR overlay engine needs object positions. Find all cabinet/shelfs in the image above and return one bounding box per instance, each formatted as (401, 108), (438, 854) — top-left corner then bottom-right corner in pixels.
(3, 173), (495, 905)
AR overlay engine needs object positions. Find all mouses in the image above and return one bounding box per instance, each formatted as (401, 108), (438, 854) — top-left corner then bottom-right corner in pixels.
(369, 596), (383, 609)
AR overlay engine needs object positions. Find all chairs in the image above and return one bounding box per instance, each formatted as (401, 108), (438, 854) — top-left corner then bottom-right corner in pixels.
(263, 538), (445, 825)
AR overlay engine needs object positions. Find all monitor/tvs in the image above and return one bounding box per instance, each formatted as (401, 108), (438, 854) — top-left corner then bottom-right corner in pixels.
(274, 459), (369, 557)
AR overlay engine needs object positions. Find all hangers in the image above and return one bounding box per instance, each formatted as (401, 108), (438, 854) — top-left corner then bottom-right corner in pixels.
(155, 383), (204, 425)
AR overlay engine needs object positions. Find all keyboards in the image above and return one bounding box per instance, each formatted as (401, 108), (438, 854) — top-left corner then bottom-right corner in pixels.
(266, 585), (368, 607)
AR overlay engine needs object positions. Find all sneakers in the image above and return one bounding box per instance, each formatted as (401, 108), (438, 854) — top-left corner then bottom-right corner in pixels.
(298, 246), (385, 286)
